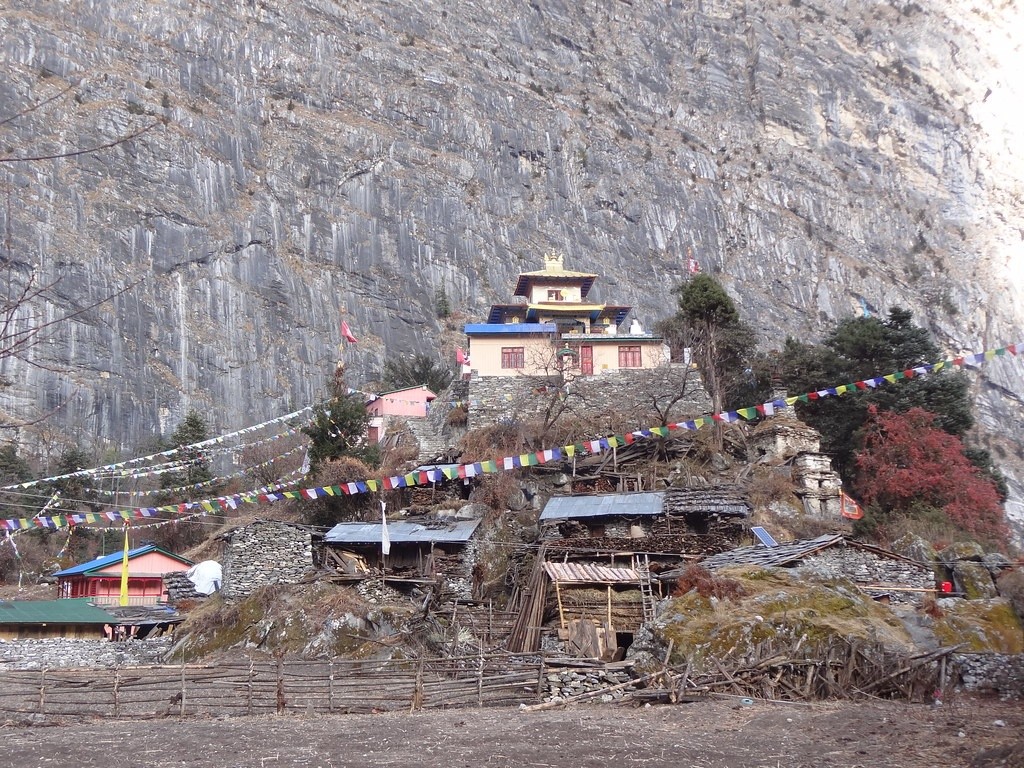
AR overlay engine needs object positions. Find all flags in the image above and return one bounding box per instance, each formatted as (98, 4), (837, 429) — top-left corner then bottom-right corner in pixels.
(86, 499), (241, 524)
(377, 445), (574, 490)
(577, 401), (773, 454)
(342, 321), (356, 342)
(244, 480), (376, 502)
(0, 514), (86, 530)
(946, 343), (1023, 369)
(773, 361), (943, 408)
